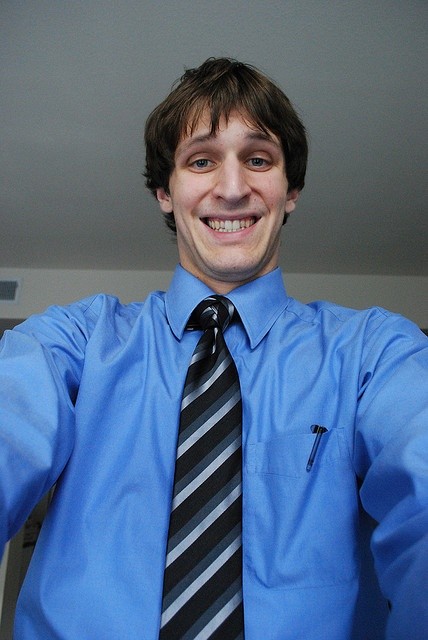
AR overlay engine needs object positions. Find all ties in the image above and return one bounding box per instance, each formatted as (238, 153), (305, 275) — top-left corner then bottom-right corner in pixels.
(159, 296), (248, 639)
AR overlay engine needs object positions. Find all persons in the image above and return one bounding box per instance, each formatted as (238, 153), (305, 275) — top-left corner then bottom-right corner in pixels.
(0, 55), (428, 640)
